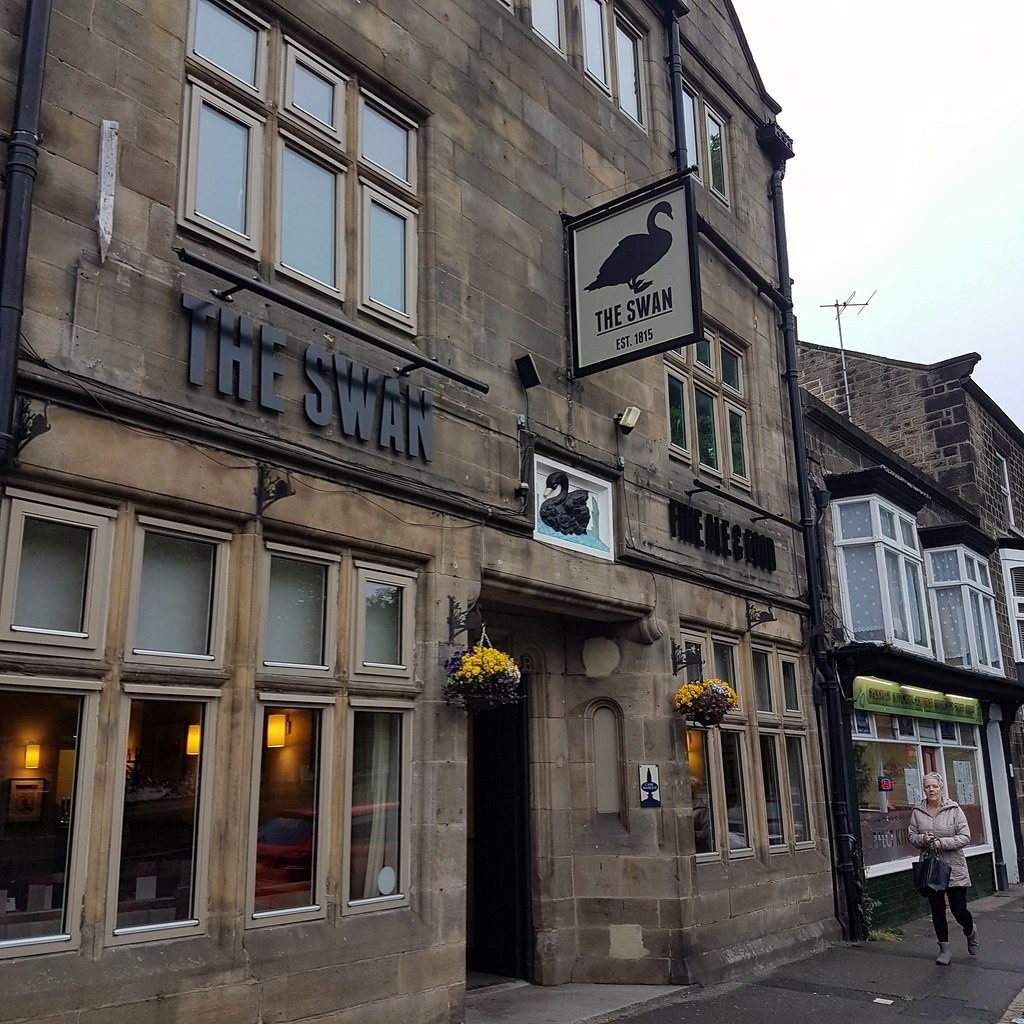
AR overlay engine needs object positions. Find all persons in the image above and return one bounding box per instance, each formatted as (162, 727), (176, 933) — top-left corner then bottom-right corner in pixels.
(908, 772), (980, 965)
(690, 776), (711, 854)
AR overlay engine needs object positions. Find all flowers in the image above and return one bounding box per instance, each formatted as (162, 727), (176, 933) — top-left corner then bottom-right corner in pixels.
(446, 649), (525, 710)
(672, 680), (737, 724)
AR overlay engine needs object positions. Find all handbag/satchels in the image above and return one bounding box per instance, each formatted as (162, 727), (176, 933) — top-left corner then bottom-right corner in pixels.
(912, 839), (952, 897)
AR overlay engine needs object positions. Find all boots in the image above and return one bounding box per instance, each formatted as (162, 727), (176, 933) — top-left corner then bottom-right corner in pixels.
(936, 941), (952, 964)
(963, 923), (980, 955)
